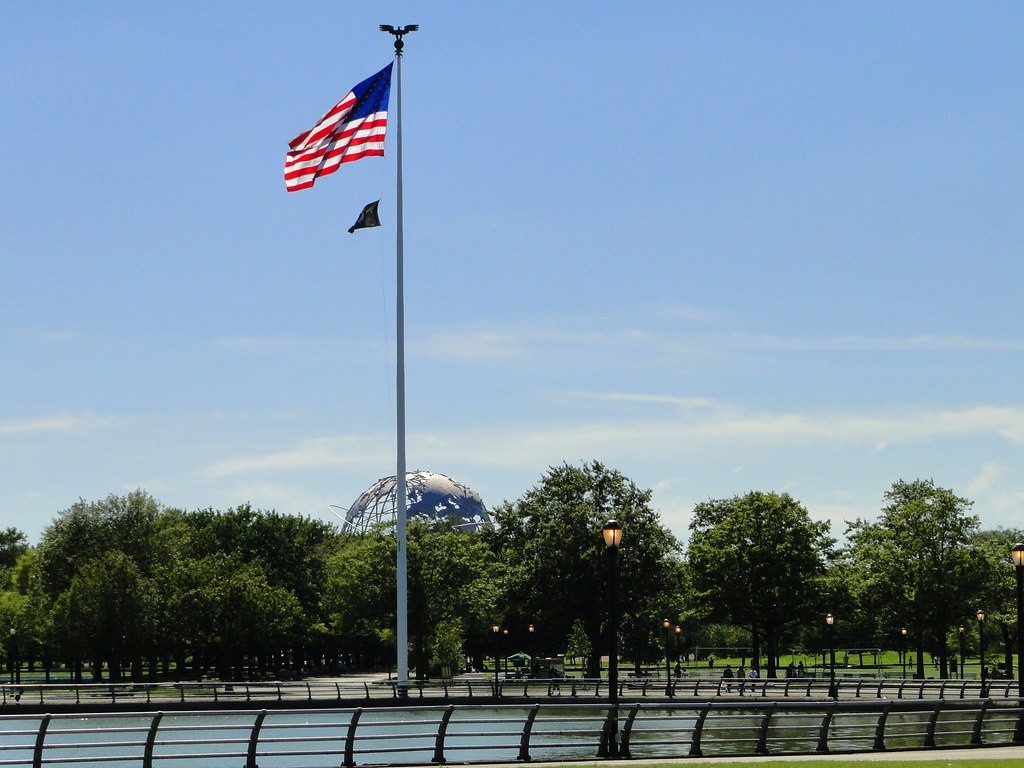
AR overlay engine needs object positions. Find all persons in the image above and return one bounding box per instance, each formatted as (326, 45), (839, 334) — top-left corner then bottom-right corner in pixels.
(785, 660), (806, 678)
(708, 657), (714, 668)
(984, 667), (1010, 679)
(933, 657), (939, 670)
(723, 665), (734, 691)
(749, 665), (758, 692)
(909, 656), (913, 669)
(843, 654), (849, 667)
(737, 665), (746, 686)
(674, 661), (687, 677)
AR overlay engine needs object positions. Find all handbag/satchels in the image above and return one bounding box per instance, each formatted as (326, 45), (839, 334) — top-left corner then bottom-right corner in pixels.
(784, 672), (787, 677)
(722, 679), (728, 687)
(799, 669), (803, 676)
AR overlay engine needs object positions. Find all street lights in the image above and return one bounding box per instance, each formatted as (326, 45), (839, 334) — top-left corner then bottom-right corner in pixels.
(529, 623), (535, 673)
(1011, 543), (1024, 742)
(959, 624), (964, 679)
(826, 613), (839, 697)
(9, 626), (16, 696)
(664, 619), (674, 694)
(492, 623), (502, 700)
(676, 626), (681, 677)
(977, 610), (988, 698)
(603, 521), (624, 757)
(902, 626), (907, 679)
(504, 627), (508, 675)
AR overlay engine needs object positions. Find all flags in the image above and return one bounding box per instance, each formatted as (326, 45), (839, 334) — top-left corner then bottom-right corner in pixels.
(283, 61), (395, 193)
(348, 200), (382, 234)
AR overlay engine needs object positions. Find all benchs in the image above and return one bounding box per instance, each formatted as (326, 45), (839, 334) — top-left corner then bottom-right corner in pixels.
(886, 672), (917, 678)
(948, 673), (977, 680)
